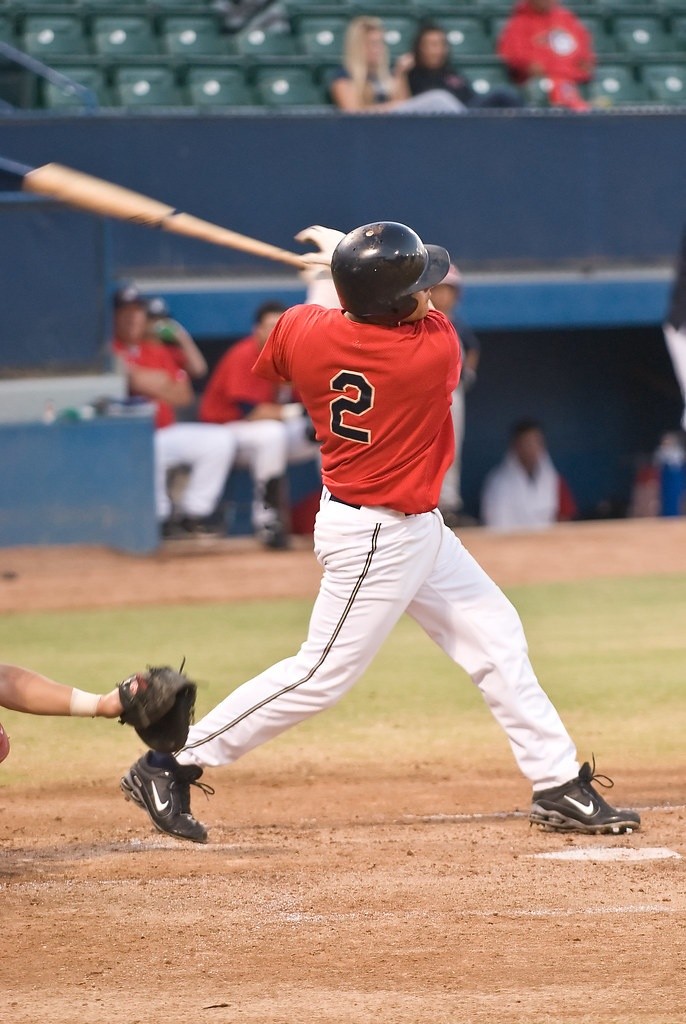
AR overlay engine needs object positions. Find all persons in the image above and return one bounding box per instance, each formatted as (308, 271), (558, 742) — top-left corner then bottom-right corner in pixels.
(112, 288), (235, 539)
(407, 24), (522, 108)
(122, 221), (640, 845)
(198, 302), (319, 549)
(479, 420), (575, 526)
(431, 264), (480, 526)
(498, 0), (595, 105)
(0, 661), (196, 763)
(330, 16), (464, 112)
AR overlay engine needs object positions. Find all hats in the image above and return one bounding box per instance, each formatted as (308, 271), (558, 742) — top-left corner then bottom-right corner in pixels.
(117, 282), (149, 309)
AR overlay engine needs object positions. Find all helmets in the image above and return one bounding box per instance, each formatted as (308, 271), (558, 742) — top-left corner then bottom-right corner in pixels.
(330, 222), (450, 323)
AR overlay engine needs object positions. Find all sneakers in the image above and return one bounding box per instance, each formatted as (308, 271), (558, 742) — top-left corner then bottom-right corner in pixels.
(120, 750), (215, 843)
(529, 752), (641, 836)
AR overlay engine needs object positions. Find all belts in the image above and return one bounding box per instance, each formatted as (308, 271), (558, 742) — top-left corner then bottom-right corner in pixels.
(330, 494), (412, 517)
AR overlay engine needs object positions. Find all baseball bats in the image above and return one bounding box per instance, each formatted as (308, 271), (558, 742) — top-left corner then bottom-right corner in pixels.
(24, 161), (307, 269)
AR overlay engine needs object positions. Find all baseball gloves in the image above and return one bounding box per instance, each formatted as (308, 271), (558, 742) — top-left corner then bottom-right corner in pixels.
(114, 654), (216, 756)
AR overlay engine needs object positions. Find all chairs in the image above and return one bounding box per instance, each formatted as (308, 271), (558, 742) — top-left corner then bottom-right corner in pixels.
(1, 0), (686, 111)
